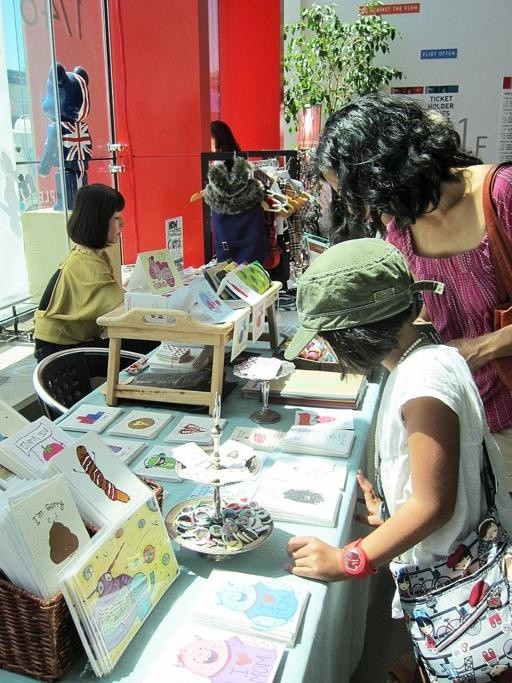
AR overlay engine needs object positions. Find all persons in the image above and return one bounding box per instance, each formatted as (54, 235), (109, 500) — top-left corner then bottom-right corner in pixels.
(32, 183), (160, 413)
(282, 238), (512, 683)
(211, 120), (241, 153)
(311, 96), (512, 541)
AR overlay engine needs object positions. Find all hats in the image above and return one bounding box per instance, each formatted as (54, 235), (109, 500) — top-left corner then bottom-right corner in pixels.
(283, 236), (444, 362)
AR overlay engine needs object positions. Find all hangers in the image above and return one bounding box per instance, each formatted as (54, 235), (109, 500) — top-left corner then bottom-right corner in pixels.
(189, 149), (312, 218)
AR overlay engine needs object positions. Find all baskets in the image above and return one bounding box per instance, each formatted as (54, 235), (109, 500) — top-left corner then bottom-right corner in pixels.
(0, 474), (165, 683)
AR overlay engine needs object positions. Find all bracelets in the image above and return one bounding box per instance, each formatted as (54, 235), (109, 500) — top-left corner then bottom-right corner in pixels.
(380, 501), (387, 521)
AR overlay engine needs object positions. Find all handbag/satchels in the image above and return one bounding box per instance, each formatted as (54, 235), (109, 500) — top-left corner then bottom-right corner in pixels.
(388, 505), (512, 683)
(483, 161), (509, 389)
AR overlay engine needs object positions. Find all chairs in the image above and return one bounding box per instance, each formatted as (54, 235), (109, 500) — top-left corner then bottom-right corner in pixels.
(32, 346), (148, 421)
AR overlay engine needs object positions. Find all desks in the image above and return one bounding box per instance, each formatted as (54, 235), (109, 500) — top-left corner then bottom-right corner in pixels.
(0, 291), (391, 683)
(94, 277), (284, 418)
